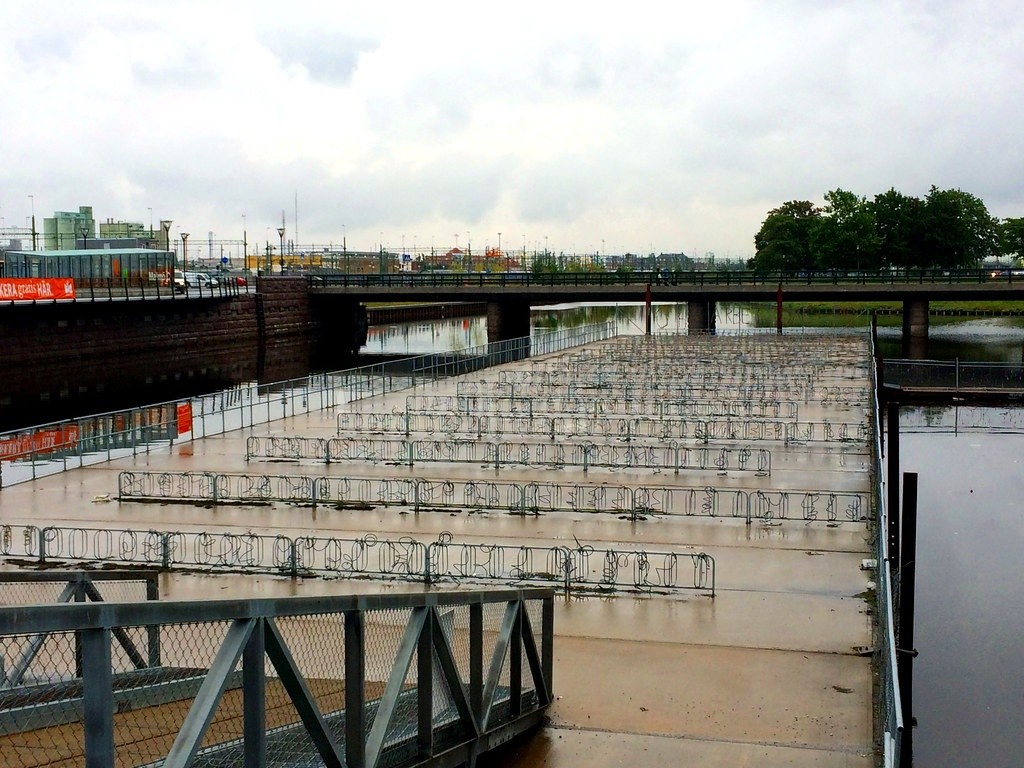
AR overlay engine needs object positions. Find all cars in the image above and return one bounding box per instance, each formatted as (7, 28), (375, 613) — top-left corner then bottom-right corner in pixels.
(156, 264), (314, 289)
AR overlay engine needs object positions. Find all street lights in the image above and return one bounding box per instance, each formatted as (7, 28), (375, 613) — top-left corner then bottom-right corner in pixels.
(342, 224), (346, 288)
(431, 235), (436, 277)
(242, 214), (249, 293)
(80, 227), (89, 249)
(522, 234), (526, 271)
(28, 194), (35, 251)
(380, 231), (384, 272)
(148, 207), (153, 239)
(498, 232), (501, 267)
(413, 236), (417, 256)
(466, 231), (471, 264)
(545, 236), (548, 265)
(180, 233), (189, 281)
(162, 221), (173, 252)
(277, 228), (286, 277)
(402, 234), (406, 255)
(455, 234), (459, 249)
